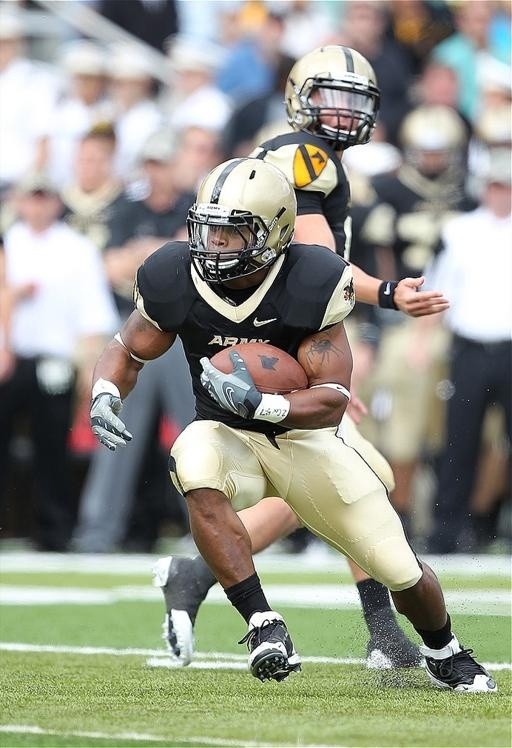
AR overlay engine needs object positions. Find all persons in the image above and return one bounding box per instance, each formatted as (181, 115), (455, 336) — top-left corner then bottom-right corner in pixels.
(1, 0), (512, 554)
(90, 156), (498, 693)
(150, 43), (449, 669)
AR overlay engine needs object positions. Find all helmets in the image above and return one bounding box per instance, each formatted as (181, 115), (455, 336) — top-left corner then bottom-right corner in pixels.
(284, 44), (381, 147)
(187, 157), (303, 282)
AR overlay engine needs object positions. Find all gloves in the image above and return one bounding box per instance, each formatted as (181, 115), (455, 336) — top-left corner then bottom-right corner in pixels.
(90, 392), (132, 450)
(200, 351), (262, 420)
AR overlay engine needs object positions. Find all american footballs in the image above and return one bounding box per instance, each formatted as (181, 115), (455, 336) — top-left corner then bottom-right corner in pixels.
(208, 343), (309, 394)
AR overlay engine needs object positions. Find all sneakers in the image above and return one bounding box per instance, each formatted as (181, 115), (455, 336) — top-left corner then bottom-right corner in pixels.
(419, 636), (498, 695)
(239, 612), (302, 682)
(366, 636), (424, 670)
(153, 554), (195, 665)
